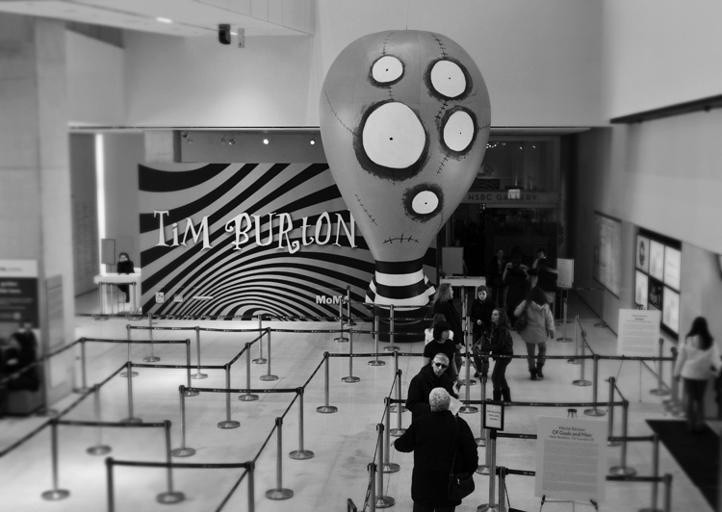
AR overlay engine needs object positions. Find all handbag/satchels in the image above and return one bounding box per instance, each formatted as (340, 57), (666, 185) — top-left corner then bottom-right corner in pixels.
(447, 416), (475, 502)
(516, 300), (531, 333)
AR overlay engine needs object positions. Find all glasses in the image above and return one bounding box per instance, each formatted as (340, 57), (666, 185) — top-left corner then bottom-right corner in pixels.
(434, 362), (447, 368)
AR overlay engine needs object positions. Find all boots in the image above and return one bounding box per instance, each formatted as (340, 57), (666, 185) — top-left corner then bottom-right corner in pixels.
(529, 362), (544, 380)
(493, 387), (511, 403)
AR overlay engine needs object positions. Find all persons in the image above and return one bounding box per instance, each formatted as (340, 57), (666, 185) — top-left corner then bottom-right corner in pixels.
(420, 246), (559, 405)
(7, 318), (41, 392)
(406, 350), (459, 425)
(672, 316), (720, 433)
(395, 386), (478, 512)
(115, 251), (135, 302)
